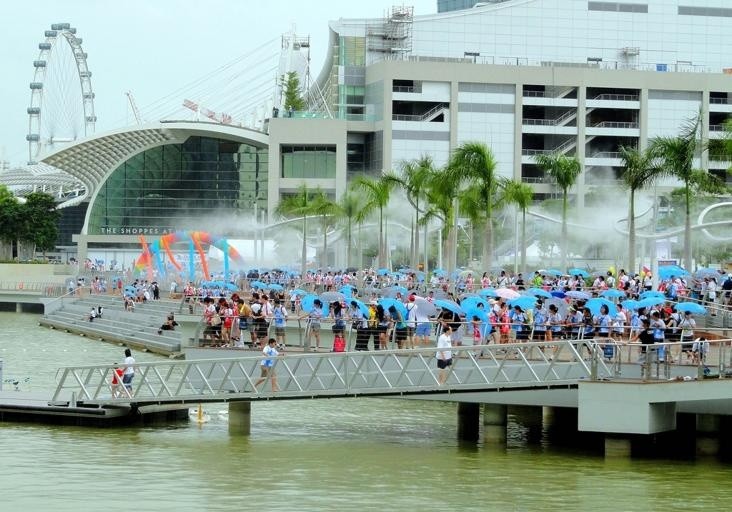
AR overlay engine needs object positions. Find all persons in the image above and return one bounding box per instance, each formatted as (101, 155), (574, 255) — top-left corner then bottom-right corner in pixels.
(156, 269), (293, 350)
(117, 348), (136, 399)
(252, 339), (284, 395)
(65, 259), (163, 324)
(298, 267), (434, 352)
(431, 269), (732, 365)
(434, 322), (455, 389)
(110, 362), (123, 400)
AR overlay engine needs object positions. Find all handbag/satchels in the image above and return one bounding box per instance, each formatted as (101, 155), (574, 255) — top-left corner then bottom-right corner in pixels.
(604, 344), (613, 358)
(333, 334), (346, 351)
(331, 319), (344, 333)
(211, 314), (222, 326)
(521, 319), (532, 335)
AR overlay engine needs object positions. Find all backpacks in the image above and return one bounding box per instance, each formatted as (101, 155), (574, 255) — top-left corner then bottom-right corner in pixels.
(671, 314), (683, 334)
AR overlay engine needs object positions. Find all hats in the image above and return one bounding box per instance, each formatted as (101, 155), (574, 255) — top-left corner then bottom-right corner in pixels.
(488, 299), (499, 305)
(408, 295), (415, 301)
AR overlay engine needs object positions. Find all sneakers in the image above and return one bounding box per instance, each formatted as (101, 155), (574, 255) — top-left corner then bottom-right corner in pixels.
(469, 348), (555, 362)
(210, 339), (265, 351)
